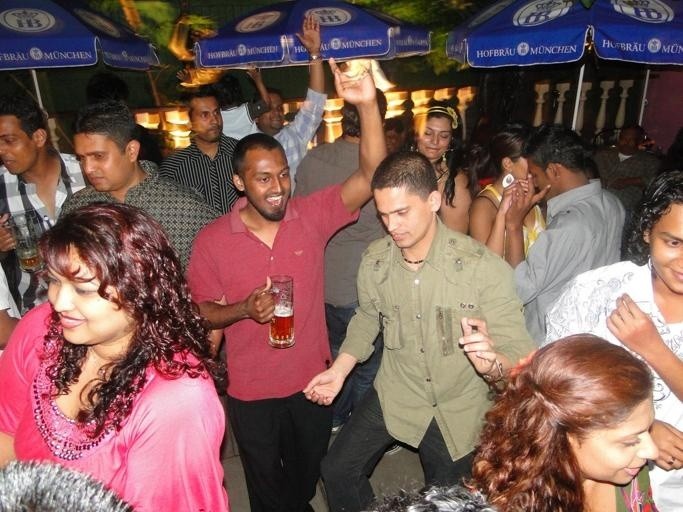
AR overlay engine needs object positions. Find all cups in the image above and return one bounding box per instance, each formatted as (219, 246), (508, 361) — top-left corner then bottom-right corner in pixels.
(1, 210), (44, 273)
(254, 274), (297, 349)
